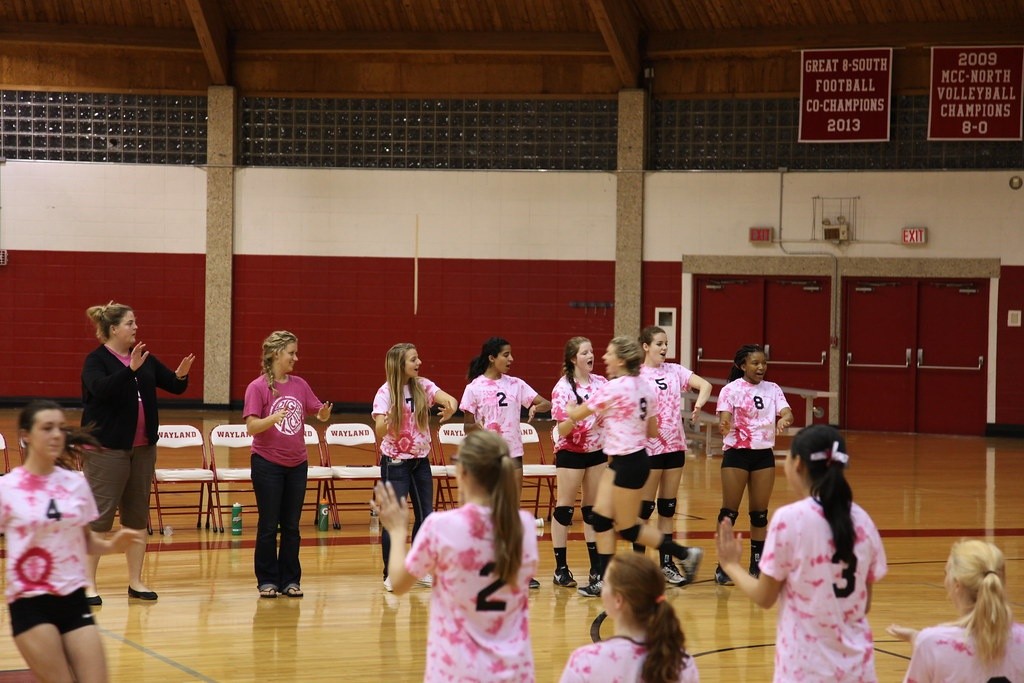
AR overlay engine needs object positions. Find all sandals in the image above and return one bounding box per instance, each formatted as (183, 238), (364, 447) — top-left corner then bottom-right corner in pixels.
(260, 587), (277, 598)
(281, 586), (303, 598)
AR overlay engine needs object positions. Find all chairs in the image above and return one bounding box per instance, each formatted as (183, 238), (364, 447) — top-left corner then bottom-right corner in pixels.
(0, 421), (565, 538)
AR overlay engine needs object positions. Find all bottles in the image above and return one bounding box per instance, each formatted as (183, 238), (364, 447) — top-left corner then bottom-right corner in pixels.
(369, 508), (380, 543)
(232, 502), (242, 535)
(318, 498), (329, 531)
(164, 525), (173, 535)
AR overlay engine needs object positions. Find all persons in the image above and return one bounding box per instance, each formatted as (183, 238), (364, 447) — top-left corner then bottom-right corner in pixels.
(552, 337), (607, 587)
(886, 540), (1024, 683)
(370, 432), (538, 683)
(558, 553), (699, 683)
(0, 401), (144, 683)
(714, 425), (888, 683)
(633, 328), (712, 585)
(459, 337), (552, 587)
(372, 344), (457, 591)
(566, 337), (659, 597)
(81, 303), (195, 604)
(715, 346), (794, 585)
(242, 331), (333, 598)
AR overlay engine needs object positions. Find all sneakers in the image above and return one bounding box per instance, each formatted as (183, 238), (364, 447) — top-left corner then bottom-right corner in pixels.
(660, 562), (687, 585)
(588, 573), (601, 584)
(578, 580), (604, 597)
(552, 565), (577, 587)
(678, 548), (702, 584)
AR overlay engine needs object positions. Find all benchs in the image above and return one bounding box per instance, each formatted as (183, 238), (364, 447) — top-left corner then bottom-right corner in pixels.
(680, 377), (838, 457)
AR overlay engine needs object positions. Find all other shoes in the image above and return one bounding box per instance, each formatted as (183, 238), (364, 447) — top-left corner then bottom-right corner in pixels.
(749, 568), (760, 578)
(715, 568), (734, 585)
(529, 579), (540, 588)
(86, 595), (102, 605)
(383, 575), (393, 592)
(417, 574), (433, 588)
(127, 585), (159, 599)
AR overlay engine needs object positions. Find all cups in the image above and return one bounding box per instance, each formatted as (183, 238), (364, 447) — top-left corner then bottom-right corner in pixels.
(536, 529), (545, 537)
(536, 517), (544, 528)
